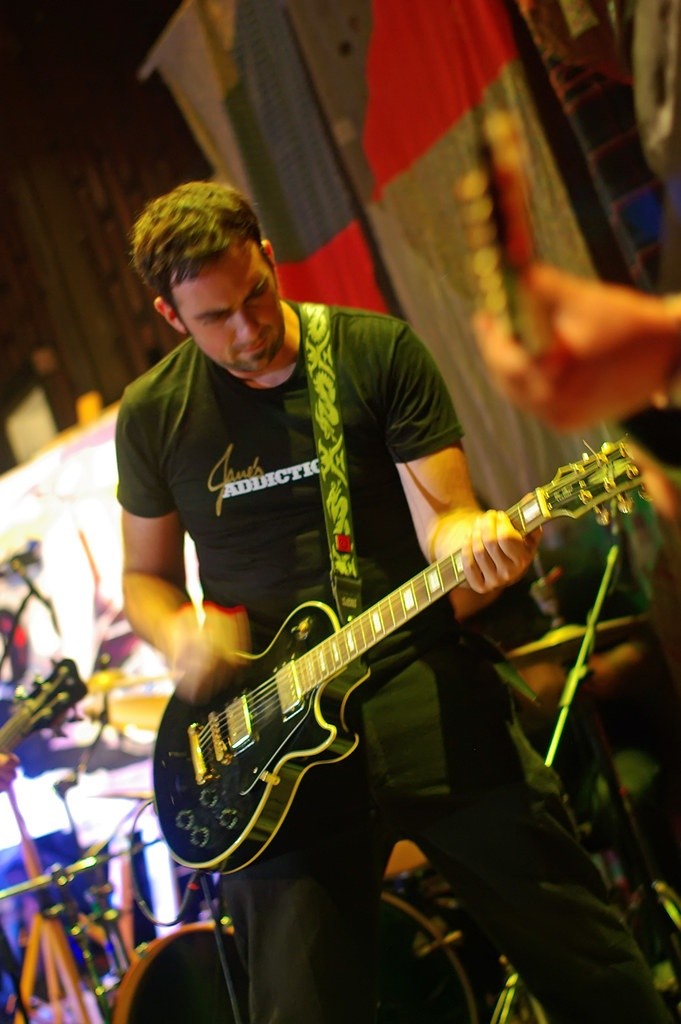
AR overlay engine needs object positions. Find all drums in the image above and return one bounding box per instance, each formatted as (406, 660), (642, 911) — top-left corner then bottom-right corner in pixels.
(111, 859), (547, 1024)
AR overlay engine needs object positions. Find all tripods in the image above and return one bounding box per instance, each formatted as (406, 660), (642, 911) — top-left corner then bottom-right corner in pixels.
(0, 754), (127, 1024)
(529, 543), (681, 988)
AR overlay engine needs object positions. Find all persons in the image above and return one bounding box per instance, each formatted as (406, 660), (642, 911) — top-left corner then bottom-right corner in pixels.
(112, 177), (681, 1024)
(469, 259), (681, 433)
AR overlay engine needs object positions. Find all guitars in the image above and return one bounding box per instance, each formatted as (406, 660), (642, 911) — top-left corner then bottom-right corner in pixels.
(0, 655), (90, 761)
(461, 116), (681, 662)
(154, 437), (643, 874)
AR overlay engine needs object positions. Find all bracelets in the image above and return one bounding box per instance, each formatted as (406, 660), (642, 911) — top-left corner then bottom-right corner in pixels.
(651, 291), (681, 410)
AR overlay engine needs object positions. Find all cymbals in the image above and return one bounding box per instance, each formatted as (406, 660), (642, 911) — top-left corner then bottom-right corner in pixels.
(501, 611), (648, 661)
